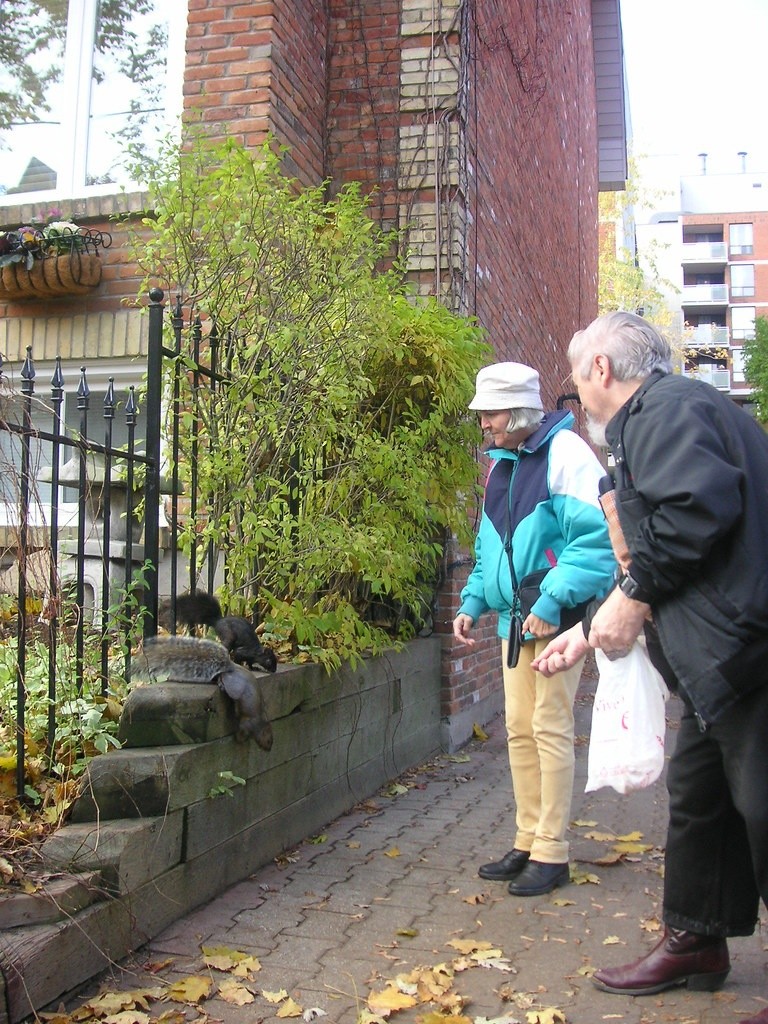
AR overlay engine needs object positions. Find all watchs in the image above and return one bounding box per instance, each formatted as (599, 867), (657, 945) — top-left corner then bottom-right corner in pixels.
(619, 571), (654, 604)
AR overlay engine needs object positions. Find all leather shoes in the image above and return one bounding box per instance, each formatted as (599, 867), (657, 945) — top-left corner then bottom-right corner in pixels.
(478, 848), (571, 896)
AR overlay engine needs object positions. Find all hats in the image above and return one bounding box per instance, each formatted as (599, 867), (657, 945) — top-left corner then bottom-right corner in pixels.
(468, 362), (544, 411)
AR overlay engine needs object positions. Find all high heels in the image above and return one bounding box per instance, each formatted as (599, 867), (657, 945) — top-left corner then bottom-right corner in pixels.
(591, 925), (732, 997)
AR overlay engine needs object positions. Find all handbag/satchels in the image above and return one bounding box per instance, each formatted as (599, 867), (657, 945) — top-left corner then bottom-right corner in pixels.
(518, 567), (588, 627)
(585, 640), (671, 794)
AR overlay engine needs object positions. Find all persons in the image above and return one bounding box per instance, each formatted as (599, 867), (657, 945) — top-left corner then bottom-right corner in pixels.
(452, 361), (621, 896)
(526, 310), (768, 994)
